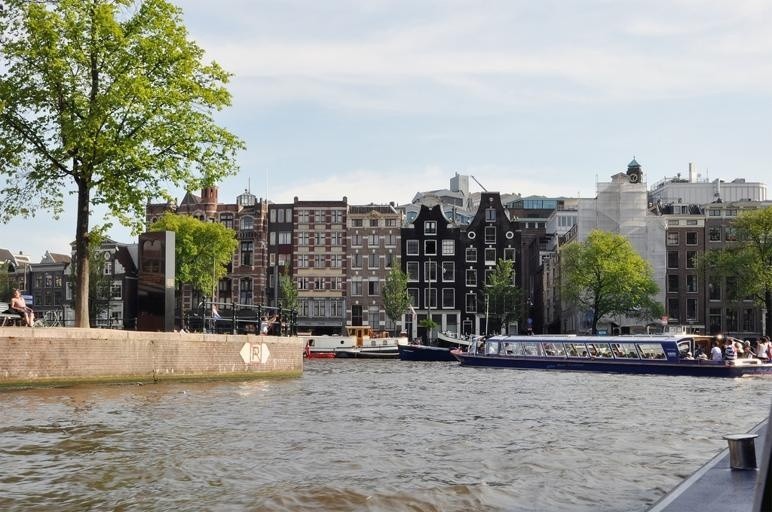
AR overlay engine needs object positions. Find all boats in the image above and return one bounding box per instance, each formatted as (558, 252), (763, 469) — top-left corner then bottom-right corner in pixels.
(306, 352), (336, 359)
(449, 333), (772, 376)
(303, 345), (400, 359)
(436, 331), (486, 352)
(397, 342), (460, 361)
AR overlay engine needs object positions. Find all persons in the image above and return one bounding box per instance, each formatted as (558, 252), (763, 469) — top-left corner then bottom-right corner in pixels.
(259, 314), (278, 336)
(477, 336), (772, 365)
(11, 288), (35, 328)
